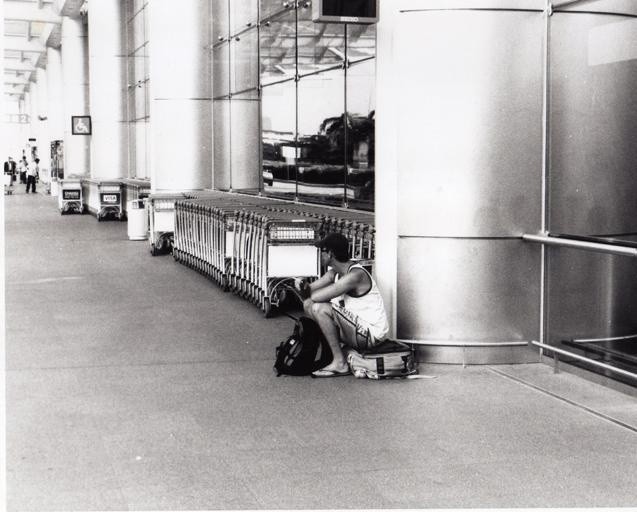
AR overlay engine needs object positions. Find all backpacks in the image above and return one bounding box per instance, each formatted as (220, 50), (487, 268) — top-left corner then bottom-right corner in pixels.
(272, 317), (330, 378)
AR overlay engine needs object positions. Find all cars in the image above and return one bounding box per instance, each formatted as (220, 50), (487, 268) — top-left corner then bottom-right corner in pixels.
(263, 170), (273, 186)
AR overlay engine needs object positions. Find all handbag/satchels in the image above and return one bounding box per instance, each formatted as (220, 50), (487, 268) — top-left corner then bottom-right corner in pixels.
(340, 326), (416, 381)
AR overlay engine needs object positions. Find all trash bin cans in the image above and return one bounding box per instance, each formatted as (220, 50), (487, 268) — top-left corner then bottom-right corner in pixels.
(127, 199), (149, 241)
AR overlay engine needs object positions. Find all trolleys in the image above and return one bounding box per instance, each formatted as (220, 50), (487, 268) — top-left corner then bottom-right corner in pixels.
(4, 172), (15, 195)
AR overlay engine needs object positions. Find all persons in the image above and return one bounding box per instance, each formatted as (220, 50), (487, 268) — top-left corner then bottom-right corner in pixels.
(295, 232), (389, 379)
(4, 155), (40, 196)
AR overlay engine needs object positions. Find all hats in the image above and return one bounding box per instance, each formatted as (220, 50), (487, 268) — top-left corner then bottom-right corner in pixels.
(308, 233), (350, 259)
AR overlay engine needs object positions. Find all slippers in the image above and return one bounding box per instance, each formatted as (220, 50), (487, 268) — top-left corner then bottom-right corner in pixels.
(308, 366), (351, 380)
